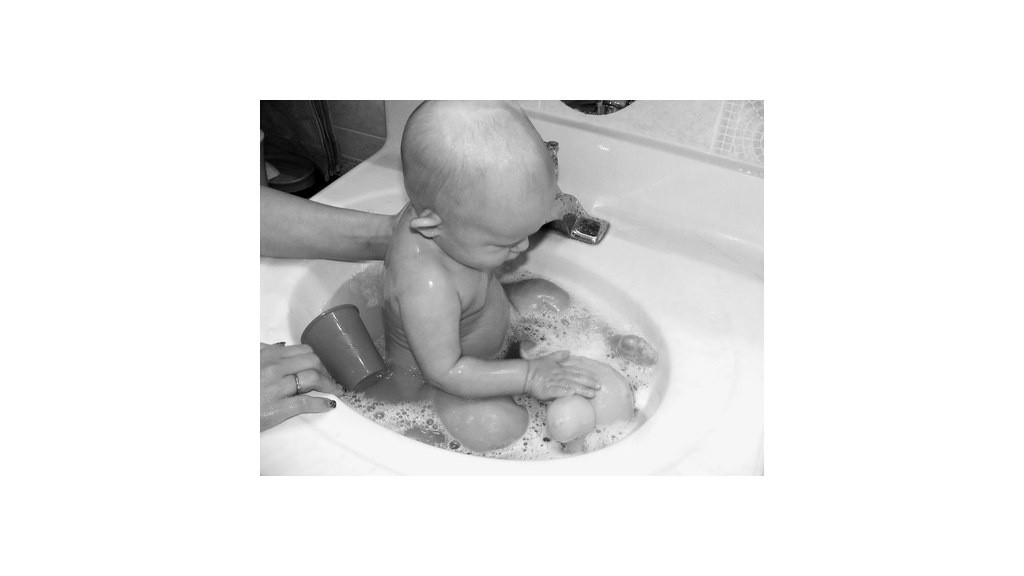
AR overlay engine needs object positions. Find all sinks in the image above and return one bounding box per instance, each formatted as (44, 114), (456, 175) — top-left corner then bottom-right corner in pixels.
(286, 240), (674, 463)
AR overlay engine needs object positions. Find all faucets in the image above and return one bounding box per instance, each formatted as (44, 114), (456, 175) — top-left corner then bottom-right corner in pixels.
(541, 140), (611, 244)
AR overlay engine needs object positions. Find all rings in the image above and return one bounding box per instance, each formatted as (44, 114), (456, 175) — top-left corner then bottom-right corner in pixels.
(292, 374), (300, 395)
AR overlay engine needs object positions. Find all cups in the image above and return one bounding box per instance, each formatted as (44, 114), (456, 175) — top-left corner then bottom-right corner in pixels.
(301, 304), (388, 392)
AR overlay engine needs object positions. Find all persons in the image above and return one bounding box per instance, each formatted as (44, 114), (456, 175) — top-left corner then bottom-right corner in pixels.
(383, 100), (634, 454)
(260, 185), (410, 433)
